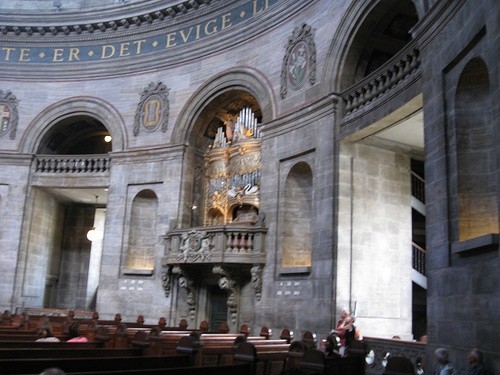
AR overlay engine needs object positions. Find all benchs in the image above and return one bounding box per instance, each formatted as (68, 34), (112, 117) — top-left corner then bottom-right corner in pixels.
(0, 310), (366, 375)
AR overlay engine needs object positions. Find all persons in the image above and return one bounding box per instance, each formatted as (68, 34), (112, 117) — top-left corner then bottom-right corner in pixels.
(332, 311), (356, 355)
(434, 348), (458, 375)
(467, 349), (488, 375)
(35, 323), (89, 343)
